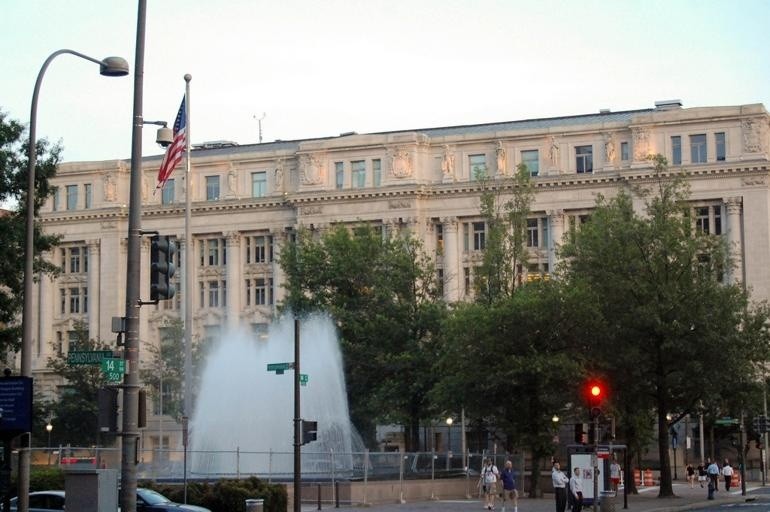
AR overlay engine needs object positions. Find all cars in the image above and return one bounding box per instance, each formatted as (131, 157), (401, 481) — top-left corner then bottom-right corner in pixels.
(117, 485), (212, 512)
(0, 489), (121, 512)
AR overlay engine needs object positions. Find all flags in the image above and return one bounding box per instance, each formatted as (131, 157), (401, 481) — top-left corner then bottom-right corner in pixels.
(156, 93), (188, 191)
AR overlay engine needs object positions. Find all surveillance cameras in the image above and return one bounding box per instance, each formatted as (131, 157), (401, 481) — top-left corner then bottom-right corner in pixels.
(155, 127), (174, 149)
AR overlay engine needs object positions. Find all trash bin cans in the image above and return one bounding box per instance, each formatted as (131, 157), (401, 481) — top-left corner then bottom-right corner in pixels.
(245, 499), (264, 512)
(600, 491), (617, 512)
(152, 447), (170, 465)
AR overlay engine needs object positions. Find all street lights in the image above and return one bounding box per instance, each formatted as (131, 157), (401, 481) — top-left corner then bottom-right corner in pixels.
(446, 416), (454, 453)
(18, 46), (131, 377)
(46, 423), (52, 465)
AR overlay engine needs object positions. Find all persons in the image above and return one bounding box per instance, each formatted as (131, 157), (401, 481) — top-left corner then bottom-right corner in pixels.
(569, 467), (583, 511)
(478, 457), (499, 510)
(552, 460), (569, 511)
(498, 460), (518, 511)
(685, 457), (734, 491)
(609, 458), (622, 497)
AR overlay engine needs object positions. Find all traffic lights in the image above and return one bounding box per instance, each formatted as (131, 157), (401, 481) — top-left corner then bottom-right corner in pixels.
(588, 381), (604, 419)
(302, 420), (318, 445)
(752, 415), (770, 449)
(149, 235), (175, 300)
